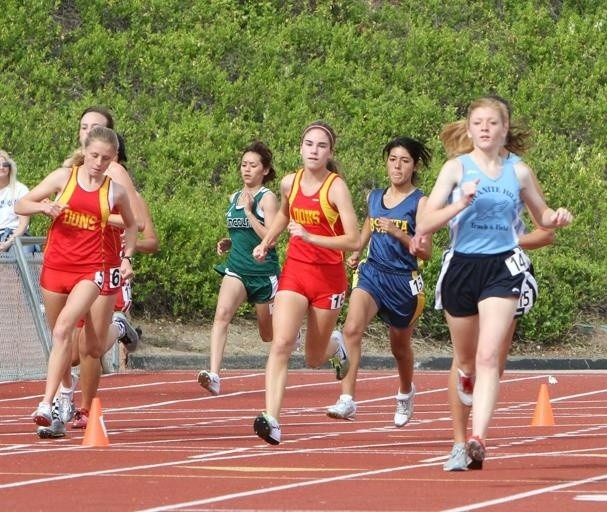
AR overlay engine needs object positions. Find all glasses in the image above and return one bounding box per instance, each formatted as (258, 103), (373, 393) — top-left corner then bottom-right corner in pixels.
(1, 163), (10, 167)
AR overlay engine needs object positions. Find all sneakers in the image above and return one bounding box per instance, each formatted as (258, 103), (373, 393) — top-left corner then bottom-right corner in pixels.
(31, 403), (52, 427)
(72, 407), (90, 429)
(444, 437), (484, 473)
(197, 370), (222, 394)
(36, 421), (66, 439)
(111, 312), (138, 350)
(252, 410), (282, 444)
(331, 330), (351, 380)
(452, 365), (475, 406)
(393, 384), (416, 427)
(59, 372), (78, 422)
(324, 391), (357, 420)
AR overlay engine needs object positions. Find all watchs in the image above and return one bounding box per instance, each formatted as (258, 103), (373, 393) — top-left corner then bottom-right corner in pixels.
(124, 256), (133, 264)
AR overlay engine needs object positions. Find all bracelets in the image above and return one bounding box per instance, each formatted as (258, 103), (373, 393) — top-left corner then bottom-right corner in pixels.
(8, 234), (15, 245)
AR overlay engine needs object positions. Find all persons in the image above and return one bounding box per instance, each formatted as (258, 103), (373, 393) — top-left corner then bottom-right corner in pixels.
(12, 128), (138, 440)
(70, 140), (160, 429)
(39, 109), (144, 419)
(415, 97), (574, 474)
(250, 119), (361, 446)
(324, 136), (433, 430)
(434, 94), (559, 408)
(194, 137), (302, 396)
(0, 148), (33, 259)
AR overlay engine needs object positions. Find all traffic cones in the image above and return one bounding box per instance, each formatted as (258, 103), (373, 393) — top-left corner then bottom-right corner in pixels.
(529, 382), (558, 428)
(75, 394), (112, 451)
